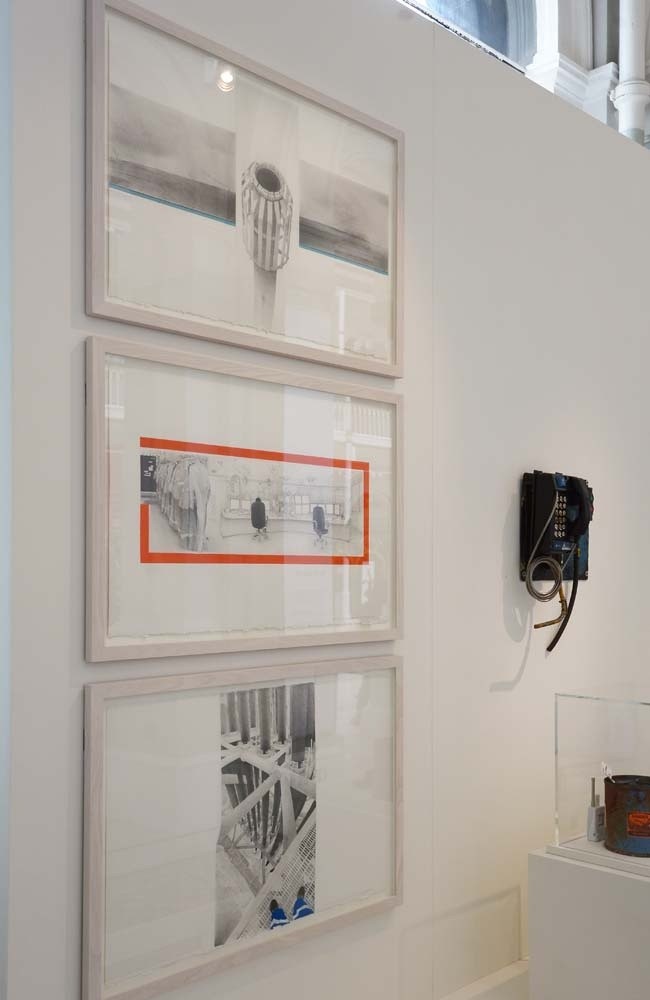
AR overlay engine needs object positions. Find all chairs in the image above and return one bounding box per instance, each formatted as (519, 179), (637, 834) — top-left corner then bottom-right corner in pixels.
(311, 507), (329, 549)
(251, 502), (269, 543)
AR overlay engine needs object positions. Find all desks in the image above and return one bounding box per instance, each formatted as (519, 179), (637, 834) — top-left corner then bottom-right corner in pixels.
(529, 848), (650, 1000)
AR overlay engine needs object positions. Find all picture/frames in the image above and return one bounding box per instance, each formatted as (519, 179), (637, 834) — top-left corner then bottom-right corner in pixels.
(86, 334), (404, 662)
(82, 0), (406, 378)
(82, 655), (403, 1000)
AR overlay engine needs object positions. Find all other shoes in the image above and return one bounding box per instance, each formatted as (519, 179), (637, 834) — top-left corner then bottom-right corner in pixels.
(270, 899), (278, 912)
(297, 887), (305, 900)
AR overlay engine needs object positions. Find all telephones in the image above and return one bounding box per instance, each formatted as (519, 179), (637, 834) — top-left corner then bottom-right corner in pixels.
(520, 470), (594, 581)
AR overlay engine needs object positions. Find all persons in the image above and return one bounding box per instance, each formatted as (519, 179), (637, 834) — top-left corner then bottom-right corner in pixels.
(269, 886), (314, 930)
